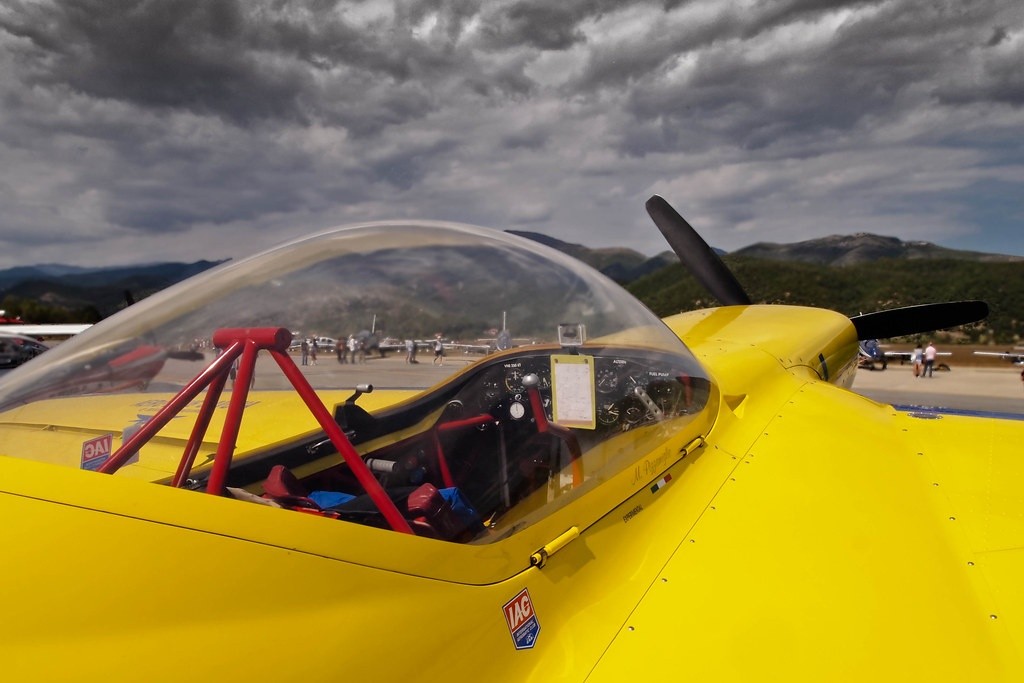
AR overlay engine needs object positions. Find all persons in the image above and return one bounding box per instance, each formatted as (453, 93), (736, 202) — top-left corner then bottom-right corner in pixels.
(229, 358), (240, 389)
(912, 344), (923, 377)
(432, 336), (444, 367)
(301, 338), (309, 365)
(250, 353), (259, 389)
(310, 339), (319, 365)
(921, 342), (937, 377)
(334, 333), (419, 364)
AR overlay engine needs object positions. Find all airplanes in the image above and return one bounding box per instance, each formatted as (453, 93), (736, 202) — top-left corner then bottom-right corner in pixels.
(0, 193), (1024, 683)
